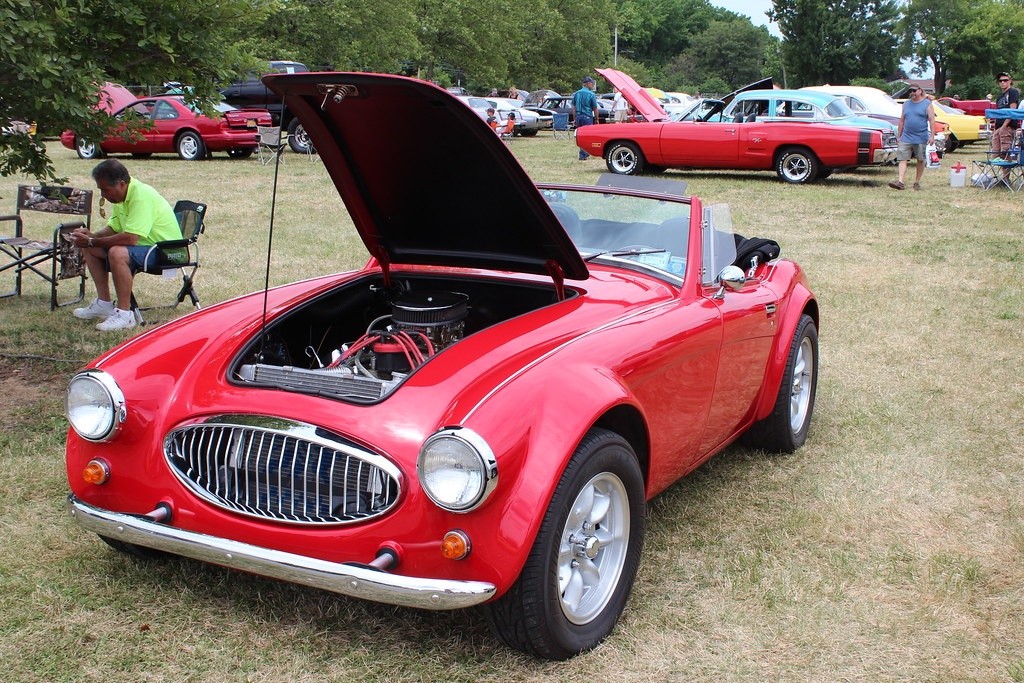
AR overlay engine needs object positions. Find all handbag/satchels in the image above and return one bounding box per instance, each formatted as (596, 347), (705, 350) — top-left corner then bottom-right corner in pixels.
(926, 143), (941, 169)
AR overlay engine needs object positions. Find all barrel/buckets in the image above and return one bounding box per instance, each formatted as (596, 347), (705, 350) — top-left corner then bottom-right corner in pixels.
(951, 160), (966, 188)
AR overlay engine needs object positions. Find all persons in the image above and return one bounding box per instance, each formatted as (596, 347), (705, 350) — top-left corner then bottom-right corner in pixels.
(991, 72), (1019, 188)
(1017, 99), (1024, 150)
(501, 113), (516, 134)
(571, 76), (599, 160)
(888, 84), (936, 190)
(68, 159), (189, 332)
(610, 86), (629, 124)
(488, 88), (499, 97)
(508, 86), (522, 100)
(485, 108), (496, 125)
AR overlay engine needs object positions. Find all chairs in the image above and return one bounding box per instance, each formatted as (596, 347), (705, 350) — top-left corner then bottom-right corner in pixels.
(549, 202), (583, 247)
(550, 113), (574, 140)
(731, 112), (756, 123)
(970, 108), (1024, 192)
(658, 217), (689, 256)
(490, 120), (515, 145)
(255, 125), (320, 166)
(0, 184), (207, 328)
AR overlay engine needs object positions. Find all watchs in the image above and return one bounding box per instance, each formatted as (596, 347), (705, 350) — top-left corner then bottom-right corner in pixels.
(88, 238), (94, 248)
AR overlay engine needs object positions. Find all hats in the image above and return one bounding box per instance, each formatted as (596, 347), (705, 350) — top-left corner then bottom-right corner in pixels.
(996, 72), (1011, 79)
(909, 83), (924, 95)
(486, 107), (495, 114)
(583, 76), (595, 84)
(507, 113), (515, 120)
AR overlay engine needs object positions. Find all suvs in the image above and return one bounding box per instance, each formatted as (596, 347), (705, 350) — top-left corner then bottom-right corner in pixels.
(220, 60), (319, 154)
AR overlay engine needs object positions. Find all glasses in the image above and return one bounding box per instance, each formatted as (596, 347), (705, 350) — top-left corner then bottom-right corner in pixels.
(908, 88), (919, 93)
(999, 78), (1010, 83)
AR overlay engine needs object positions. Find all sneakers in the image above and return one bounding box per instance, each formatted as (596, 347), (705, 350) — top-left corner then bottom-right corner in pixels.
(72, 298), (116, 320)
(94, 308), (137, 332)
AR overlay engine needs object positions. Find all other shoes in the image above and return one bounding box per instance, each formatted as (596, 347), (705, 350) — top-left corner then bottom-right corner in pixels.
(998, 180), (1011, 188)
(578, 155), (588, 161)
(889, 180), (905, 189)
(912, 182), (921, 190)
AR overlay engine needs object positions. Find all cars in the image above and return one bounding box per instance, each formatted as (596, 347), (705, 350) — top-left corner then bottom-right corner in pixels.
(61, 80), (273, 161)
(444, 75), (992, 184)
(63, 71), (820, 657)
